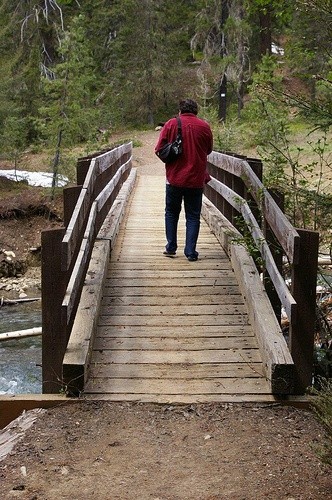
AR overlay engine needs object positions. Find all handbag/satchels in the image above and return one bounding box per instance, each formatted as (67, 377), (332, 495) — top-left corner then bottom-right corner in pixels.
(155, 116), (183, 167)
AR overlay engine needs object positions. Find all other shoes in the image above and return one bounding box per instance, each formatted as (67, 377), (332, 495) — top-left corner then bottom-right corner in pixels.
(189, 257), (192, 257)
(162, 249), (176, 256)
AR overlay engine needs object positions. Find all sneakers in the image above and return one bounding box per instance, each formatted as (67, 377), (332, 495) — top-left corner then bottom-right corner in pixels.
(187, 251), (198, 261)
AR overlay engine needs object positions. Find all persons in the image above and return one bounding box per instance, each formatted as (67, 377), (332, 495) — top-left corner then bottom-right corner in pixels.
(155, 97), (213, 261)
